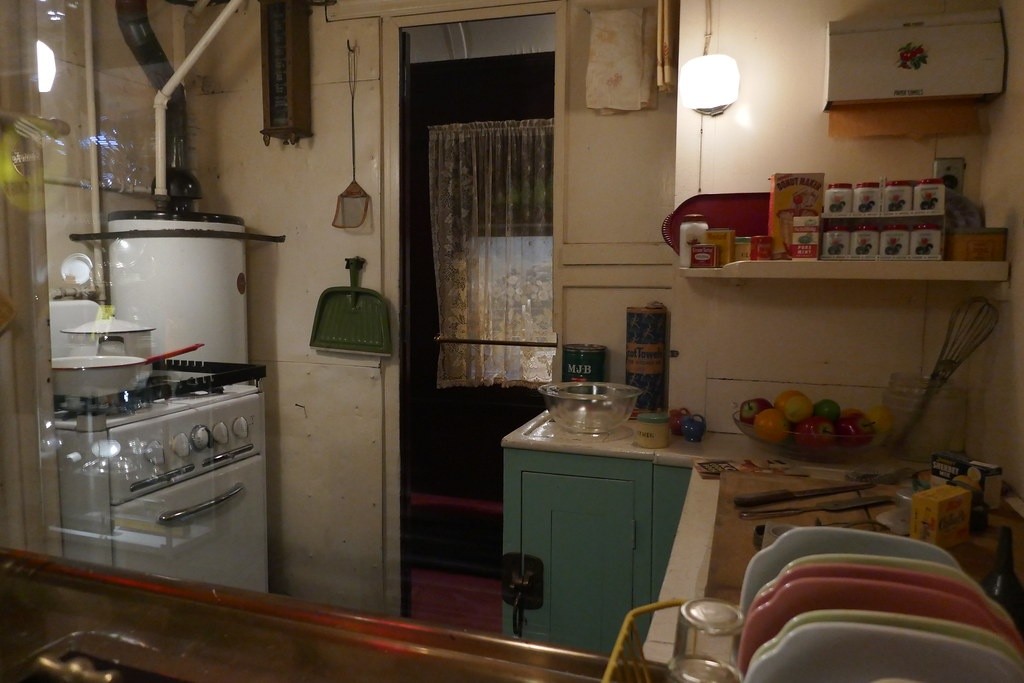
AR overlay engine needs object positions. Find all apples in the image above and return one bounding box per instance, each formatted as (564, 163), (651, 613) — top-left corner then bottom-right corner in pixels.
(738, 390), (877, 457)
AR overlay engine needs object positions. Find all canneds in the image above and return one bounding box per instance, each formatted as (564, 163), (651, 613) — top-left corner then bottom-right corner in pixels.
(561, 343), (606, 394)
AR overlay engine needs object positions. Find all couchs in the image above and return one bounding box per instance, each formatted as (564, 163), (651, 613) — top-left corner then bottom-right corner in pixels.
(407, 376), (549, 580)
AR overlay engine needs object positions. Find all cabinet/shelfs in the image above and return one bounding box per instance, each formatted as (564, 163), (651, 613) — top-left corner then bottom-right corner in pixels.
(502, 447), (693, 661)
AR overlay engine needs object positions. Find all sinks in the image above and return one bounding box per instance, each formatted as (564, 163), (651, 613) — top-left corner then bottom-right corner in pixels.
(6, 627), (201, 683)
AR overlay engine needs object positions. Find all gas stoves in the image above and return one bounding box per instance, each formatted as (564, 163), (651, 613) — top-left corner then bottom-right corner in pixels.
(54, 375), (258, 426)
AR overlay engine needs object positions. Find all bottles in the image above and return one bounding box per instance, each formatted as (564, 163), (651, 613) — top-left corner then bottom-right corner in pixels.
(980, 526), (1024, 642)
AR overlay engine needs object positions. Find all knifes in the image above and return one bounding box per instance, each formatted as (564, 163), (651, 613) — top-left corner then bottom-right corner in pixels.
(740, 494), (890, 520)
(734, 483), (873, 506)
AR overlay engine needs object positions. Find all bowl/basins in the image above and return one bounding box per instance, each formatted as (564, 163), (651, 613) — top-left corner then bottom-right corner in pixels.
(539, 382), (641, 433)
(731, 411), (891, 462)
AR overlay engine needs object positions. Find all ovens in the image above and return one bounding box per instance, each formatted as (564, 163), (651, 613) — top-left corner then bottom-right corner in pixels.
(108, 393), (269, 594)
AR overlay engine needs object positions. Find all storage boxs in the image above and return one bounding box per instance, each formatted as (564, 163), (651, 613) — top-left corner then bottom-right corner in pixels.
(770, 173), (824, 261)
(690, 226), (752, 269)
(911, 485), (971, 549)
(946, 227), (1008, 261)
(931, 452), (1002, 511)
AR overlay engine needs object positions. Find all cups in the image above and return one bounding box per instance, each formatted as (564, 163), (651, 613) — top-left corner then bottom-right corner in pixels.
(886, 375), (968, 460)
(682, 414), (705, 441)
(667, 598), (746, 683)
(895, 488), (917, 535)
(670, 408), (690, 435)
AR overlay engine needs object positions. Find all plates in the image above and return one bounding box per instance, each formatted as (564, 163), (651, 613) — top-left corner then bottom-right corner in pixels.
(749, 609), (1024, 665)
(752, 564), (1024, 650)
(737, 577), (998, 674)
(746, 621), (1024, 683)
(754, 554), (1011, 620)
(739, 526), (962, 616)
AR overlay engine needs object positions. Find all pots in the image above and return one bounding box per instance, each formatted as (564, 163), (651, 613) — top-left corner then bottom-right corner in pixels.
(52, 343), (204, 395)
(60, 316), (155, 381)
